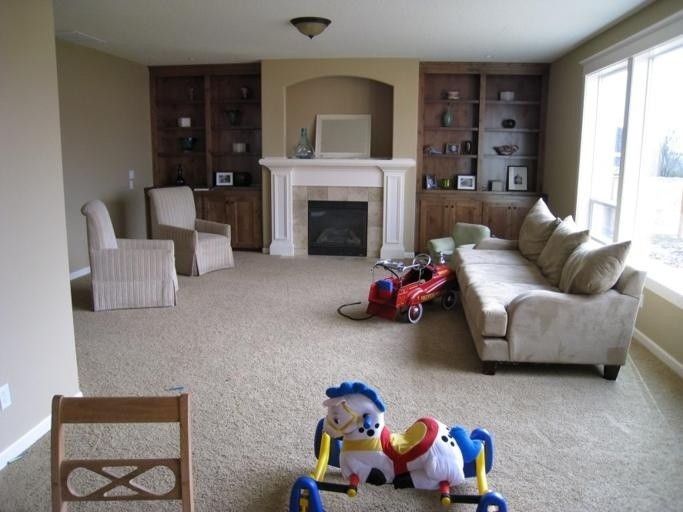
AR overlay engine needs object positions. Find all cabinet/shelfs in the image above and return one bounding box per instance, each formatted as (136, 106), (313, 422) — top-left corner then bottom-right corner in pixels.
(147, 60), (270, 254)
(418, 59), (551, 252)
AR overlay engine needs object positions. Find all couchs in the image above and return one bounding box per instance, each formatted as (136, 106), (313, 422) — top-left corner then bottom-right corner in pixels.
(456, 237), (646, 383)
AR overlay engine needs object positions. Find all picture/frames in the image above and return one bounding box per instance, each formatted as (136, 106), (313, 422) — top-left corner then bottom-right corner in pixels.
(292, 126), (313, 159)
(456, 174), (475, 190)
(215, 171), (235, 188)
(314, 114), (371, 157)
(508, 165), (528, 191)
(446, 143), (461, 156)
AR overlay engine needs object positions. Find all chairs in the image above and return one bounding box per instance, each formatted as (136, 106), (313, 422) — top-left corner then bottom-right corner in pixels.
(146, 185), (233, 275)
(426, 221), (493, 266)
(80, 199), (178, 311)
(49, 393), (195, 511)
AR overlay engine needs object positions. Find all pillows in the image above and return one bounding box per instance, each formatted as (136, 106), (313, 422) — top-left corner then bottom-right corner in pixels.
(536, 214), (589, 285)
(558, 240), (632, 295)
(517, 196), (560, 261)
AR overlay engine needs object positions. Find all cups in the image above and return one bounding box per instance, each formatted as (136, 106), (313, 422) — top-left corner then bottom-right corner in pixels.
(441, 178), (451, 189)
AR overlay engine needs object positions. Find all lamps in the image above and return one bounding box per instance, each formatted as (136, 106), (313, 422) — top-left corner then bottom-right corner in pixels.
(288, 16), (331, 38)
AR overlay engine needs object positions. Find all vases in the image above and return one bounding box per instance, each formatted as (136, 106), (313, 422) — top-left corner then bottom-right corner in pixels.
(443, 106), (455, 128)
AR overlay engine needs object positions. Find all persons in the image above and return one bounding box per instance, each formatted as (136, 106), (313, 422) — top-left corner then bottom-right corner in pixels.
(514, 173), (521, 184)
